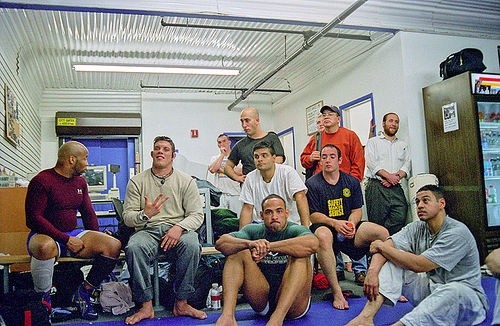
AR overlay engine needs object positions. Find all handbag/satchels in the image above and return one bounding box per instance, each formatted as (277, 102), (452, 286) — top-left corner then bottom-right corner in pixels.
(191, 176), (222, 207)
(187, 254), (228, 310)
(440, 48), (487, 80)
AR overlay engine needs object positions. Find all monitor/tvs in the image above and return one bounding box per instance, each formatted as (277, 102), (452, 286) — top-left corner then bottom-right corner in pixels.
(83, 165), (107, 193)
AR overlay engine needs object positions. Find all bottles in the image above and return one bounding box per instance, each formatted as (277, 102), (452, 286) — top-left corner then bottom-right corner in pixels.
(481, 130), (500, 148)
(485, 185), (497, 203)
(211, 283), (221, 309)
(337, 224), (353, 241)
(483, 156), (500, 177)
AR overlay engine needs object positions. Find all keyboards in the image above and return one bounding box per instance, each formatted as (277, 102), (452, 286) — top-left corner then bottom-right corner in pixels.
(89, 195), (106, 200)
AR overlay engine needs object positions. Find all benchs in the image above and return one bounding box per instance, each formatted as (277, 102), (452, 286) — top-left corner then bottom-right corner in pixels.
(56, 246), (221, 311)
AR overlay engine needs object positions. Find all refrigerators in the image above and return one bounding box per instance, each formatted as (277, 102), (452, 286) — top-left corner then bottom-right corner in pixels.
(421, 71), (500, 267)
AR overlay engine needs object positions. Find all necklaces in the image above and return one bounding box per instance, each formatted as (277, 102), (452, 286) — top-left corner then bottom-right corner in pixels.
(151, 167), (173, 185)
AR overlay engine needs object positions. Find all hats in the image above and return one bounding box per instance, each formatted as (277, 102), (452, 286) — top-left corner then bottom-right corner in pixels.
(320, 105), (341, 116)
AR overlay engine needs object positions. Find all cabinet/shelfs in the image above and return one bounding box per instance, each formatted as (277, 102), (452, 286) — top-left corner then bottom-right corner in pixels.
(423, 71), (500, 265)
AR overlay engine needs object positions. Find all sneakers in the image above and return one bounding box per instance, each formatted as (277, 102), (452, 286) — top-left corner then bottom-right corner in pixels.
(33, 288), (52, 326)
(72, 282), (98, 320)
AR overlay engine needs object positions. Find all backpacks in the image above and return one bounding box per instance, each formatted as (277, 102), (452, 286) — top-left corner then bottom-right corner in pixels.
(302, 131), (321, 180)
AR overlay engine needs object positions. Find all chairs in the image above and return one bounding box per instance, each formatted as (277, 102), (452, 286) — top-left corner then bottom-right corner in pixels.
(0, 186), (32, 294)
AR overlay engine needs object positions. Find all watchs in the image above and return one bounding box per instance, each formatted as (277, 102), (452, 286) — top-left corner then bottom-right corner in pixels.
(142, 213), (150, 221)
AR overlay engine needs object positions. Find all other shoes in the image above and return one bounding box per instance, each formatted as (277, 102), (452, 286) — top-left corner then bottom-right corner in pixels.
(355, 271), (366, 283)
(336, 271), (346, 281)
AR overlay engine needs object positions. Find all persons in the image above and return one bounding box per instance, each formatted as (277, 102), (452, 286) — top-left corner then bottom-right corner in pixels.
(300, 105), (365, 183)
(208, 134), (243, 219)
(215, 194), (319, 326)
(304, 144), (408, 310)
(345, 184), (490, 326)
(486, 248), (500, 326)
(225, 107), (286, 188)
(317, 113), (325, 131)
(368, 112), (411, 236)
(24, 141), (120, 326)
(239, 141), (311, 228)
(123, 137), (207, 325)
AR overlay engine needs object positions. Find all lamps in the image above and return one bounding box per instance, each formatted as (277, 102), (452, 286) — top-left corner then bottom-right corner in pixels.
(72, 64), (239, 76)
